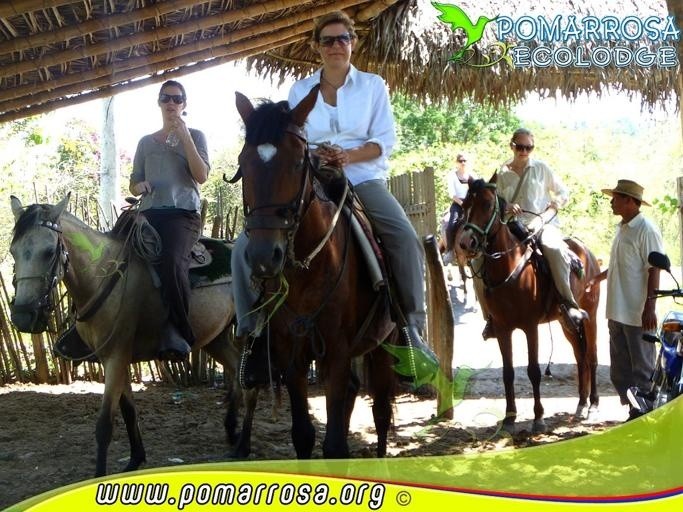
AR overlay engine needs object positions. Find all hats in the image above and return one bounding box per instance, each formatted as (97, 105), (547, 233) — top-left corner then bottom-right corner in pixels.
(600, 178), (654, 209)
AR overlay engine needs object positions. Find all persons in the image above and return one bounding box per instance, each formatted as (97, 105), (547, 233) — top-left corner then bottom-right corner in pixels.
(481, 128), (586, 339)
(55, 79), (210, 364)
(229, 9), (440, 392)
(586, 180), (667, 422)
(442, 151), (480, 265)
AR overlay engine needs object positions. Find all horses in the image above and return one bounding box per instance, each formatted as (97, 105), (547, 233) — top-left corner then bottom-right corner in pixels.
(454, 168), (600, 432)
(8, 191), (261, 478)
(234, 83), (399, 459)
(438, 212), (481, 312)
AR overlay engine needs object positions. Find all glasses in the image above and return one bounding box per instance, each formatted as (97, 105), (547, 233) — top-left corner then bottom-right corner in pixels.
(159, 93), (184, 104)
(316, 32), (351, 46)
(512, 144), (536, 153)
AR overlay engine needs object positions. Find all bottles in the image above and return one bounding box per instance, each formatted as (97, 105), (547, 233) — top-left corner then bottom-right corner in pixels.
(165, 120), (181, 148)
